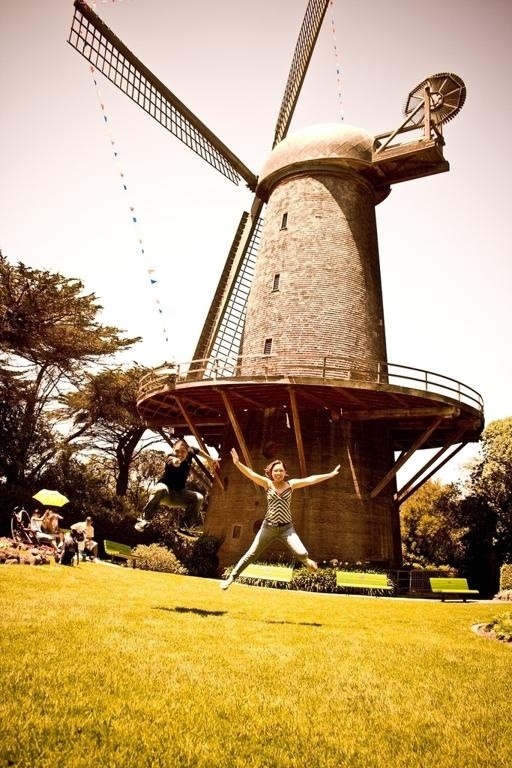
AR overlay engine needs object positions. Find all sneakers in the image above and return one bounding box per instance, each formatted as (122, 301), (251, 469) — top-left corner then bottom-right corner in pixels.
(305, 559), (317, 572)
(221, 579), (231, 589)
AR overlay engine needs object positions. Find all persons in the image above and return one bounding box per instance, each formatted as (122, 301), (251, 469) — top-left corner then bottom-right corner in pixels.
(220, 446), (341, 590)
(134, 439), (220, 541)
(30, 508), (96, 566)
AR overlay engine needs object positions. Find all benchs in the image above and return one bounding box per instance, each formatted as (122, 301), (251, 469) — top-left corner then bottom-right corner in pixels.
(104, 540), (132, 563)
(429, 577), (480, 602)
(336, 572), (393, 597)
(239, 563), (294, 583)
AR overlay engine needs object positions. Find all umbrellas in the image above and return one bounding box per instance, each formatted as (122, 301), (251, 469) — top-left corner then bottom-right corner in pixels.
(31, 488), (70, 508)
